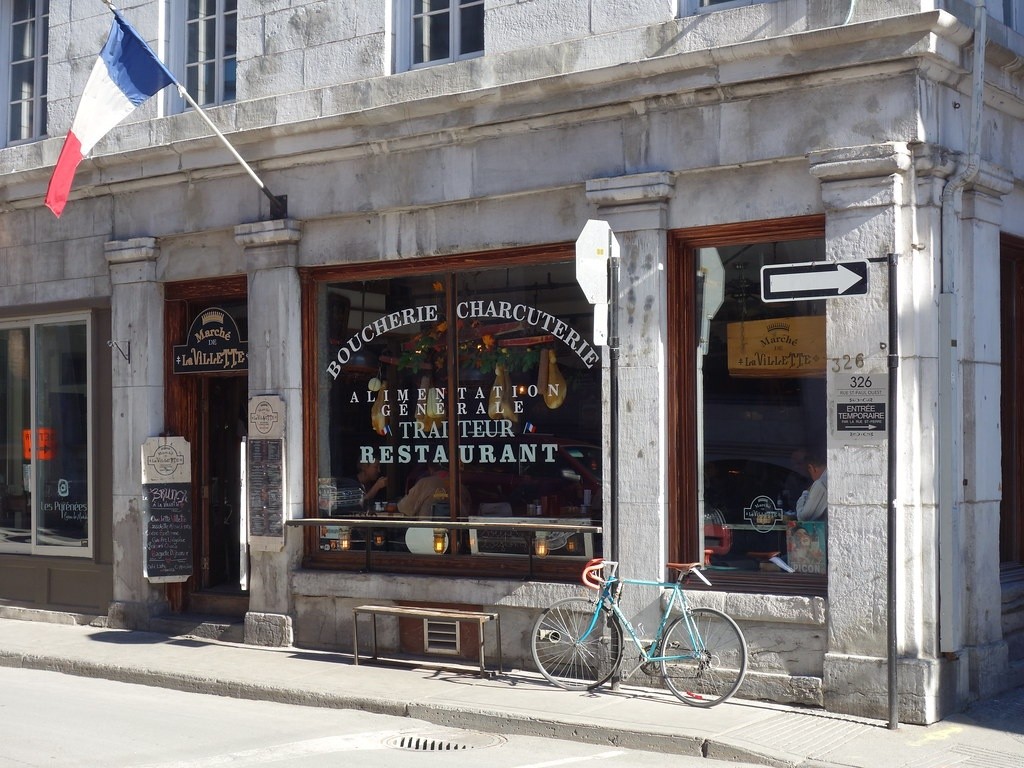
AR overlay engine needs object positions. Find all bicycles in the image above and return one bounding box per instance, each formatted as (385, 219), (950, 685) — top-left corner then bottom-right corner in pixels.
(530, 558), (750, 708)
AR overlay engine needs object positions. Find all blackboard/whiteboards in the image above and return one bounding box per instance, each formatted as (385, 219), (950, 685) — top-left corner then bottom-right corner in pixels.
(248, 394), (286, 552)
(140, 435), (193, 584)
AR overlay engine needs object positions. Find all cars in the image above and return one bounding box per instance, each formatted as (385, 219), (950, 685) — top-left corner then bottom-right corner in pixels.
(504, 428), (821, 526)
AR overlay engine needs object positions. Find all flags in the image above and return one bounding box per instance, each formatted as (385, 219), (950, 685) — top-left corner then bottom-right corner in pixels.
(43, 7), (180, 218)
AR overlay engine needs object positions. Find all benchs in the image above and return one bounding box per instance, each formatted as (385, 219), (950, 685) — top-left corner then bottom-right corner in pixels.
(352, 604), (504, 681)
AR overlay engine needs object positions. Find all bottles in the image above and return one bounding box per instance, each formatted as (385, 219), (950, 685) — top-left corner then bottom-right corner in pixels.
(525, 496), (543, 515)
(373, 501), (389, 512)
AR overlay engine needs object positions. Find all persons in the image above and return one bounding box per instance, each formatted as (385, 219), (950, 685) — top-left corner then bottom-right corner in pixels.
(396, 452), (471, 521)
(329, 454), (389, 516)
(797, 450), (829, 523)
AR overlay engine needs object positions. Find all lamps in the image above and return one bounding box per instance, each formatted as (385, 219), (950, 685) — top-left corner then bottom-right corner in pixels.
(338, 529), (350, 551)
(374, 527), (387, 551)
(535, 534), (550, 559)
(565, 532), (578, 553)
(433, 528), (447, 553)
